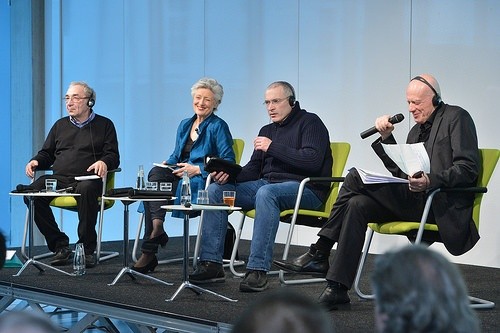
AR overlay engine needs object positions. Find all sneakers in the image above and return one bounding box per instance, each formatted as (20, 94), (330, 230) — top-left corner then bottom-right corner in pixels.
(188, 261), (225, 283)
(240, 269), (269, 292)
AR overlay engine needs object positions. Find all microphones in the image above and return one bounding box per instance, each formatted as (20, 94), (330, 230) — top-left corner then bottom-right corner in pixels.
(127, 189), (174, 196)
(360, 113), (404, 140)
(16, 184), (34, 191)
(107, 186), (133, 196)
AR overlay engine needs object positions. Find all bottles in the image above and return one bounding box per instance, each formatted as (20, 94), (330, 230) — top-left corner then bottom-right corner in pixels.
(73, 243), (85, 275)
(180, 171), (191, 205)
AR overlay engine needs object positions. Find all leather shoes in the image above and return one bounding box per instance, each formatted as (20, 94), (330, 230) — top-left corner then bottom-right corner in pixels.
(315, 283), (353, 311)
(273, 244), (330, 276)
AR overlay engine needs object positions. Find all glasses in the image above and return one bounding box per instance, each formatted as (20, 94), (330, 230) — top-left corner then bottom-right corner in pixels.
(62, 95), (88, 102)
(262, 96), (292, 107)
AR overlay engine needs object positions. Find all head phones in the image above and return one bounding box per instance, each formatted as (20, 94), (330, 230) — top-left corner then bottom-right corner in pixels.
(410, 76), (442, 107)
(86, 90), (95, 107)
(279, 82), (296, 107)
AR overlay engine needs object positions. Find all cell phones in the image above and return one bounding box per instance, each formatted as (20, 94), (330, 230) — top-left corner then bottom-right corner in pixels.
(412, 170), (425, 180)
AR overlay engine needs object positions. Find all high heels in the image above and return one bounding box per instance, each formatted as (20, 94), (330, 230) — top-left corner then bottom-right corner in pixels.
(130, 255), (158, 274)
(141, 232), (169, 248)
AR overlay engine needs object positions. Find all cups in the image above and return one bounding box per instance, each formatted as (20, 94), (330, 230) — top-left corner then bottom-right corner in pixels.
(145, 182), (158, 191)
(45, 179), (57, 193)
(160, 182), (172, 192)
(197, 190), (209, 205)
(223, 191), (236, 207)
(136, 165), (146, 189)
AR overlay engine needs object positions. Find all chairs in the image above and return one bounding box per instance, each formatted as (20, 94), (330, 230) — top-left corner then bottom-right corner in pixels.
(130, 139), (248, 269)
(353, 148), (500, 310)
(229, 141), (351, 277)
(30, 170), (120, 265)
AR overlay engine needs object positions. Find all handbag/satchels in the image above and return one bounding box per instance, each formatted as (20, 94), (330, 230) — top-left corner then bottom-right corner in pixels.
(224, 222), (239, 260)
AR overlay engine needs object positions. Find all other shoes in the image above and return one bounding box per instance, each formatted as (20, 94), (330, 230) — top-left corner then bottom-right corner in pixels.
(49, 246), (73, 262)
(85, 255), (96, 268)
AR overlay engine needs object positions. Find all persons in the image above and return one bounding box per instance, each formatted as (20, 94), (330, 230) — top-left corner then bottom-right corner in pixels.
(24, 82), (121, 268)
(234, 288), (331, 333)
(186, 80), (333, 291)
(0, 310), (60, 333)
(371, 246), (481, 333)
(132, 77), (236, 273)
(273, 74), (481, 311)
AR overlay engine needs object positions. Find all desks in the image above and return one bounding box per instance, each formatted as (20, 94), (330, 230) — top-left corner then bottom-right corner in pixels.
(160, 204), (244, 302)
(102, 196), (181, 287)
(8, 192), (81, 278)
